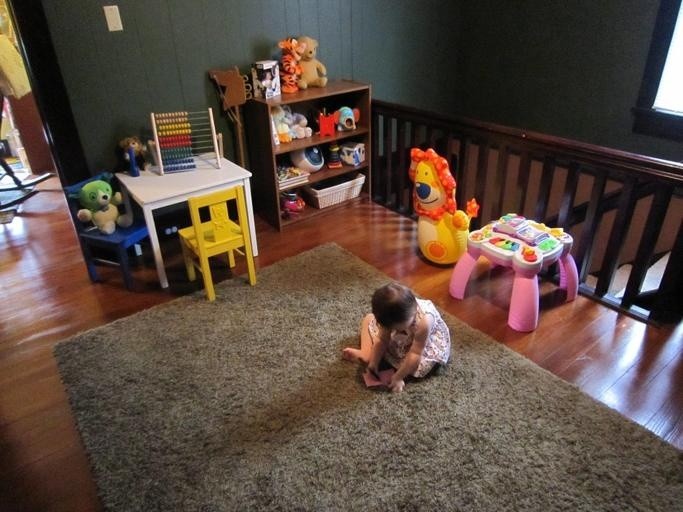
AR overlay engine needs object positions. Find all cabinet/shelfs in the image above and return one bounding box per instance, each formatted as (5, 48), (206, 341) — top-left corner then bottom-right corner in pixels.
(242, 79), (374, 230)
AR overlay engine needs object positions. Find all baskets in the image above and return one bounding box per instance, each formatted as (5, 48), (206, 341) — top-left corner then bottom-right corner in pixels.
(302, 171), (365, 210)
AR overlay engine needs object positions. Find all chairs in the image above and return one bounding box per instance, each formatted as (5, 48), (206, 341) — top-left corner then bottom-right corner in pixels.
(177, 186), (257, 301)
(63, 172), (147, 291)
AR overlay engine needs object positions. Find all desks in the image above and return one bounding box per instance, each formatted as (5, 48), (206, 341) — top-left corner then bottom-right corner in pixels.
(448, 213), (580, 332)
(117, 153), (259, 289)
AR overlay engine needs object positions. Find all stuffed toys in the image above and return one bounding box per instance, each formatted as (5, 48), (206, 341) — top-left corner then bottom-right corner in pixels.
(272, 103), (365, 221)
(77, 180), (133, 234)
(120, 136), (151, 171)
(278, 38), (306, 93)
(296, 36), (328, 89)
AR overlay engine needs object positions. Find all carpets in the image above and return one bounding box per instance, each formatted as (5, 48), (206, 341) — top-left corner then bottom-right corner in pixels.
(52, 244), (683, 511)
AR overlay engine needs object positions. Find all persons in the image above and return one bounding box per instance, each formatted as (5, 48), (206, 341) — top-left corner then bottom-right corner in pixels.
(342, 282), (450, 393)
